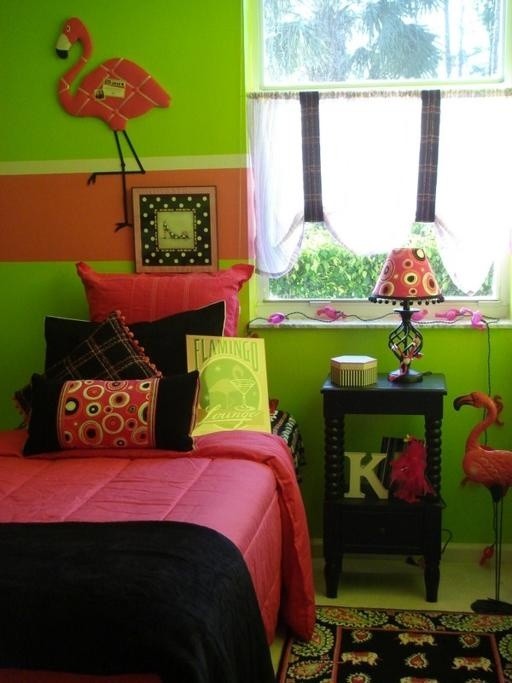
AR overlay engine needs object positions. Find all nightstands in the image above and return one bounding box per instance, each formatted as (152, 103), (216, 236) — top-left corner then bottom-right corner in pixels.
(320, 371), (447, 602)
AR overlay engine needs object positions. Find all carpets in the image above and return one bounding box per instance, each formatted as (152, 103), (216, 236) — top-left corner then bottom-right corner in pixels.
(276, 603), (512, 683)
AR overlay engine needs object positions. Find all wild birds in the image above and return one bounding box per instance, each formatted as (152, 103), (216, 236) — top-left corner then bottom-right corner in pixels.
(452, 389), (511, 604)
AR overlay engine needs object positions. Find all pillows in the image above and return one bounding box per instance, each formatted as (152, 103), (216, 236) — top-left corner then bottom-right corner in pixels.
(76, 261), (254, 336)
(21, 370), (198, 453)
(44, 299), (226, 375)
(14, 309), (165, 423)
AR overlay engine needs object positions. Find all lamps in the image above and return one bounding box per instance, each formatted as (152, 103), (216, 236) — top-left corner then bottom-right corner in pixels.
(368, 248), (446, 385)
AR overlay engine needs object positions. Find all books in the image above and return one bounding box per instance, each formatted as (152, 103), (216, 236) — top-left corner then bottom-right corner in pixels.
(186, 334), (271, 437)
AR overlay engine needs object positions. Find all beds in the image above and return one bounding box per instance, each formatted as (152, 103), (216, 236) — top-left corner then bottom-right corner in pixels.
(1, 411), (316, 683)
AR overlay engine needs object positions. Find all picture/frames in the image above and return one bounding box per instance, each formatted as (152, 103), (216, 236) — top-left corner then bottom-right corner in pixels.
(131, 185), (219, 274)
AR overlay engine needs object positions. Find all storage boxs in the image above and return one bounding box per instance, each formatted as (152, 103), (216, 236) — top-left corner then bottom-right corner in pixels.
(331, 355), (378, 387)
